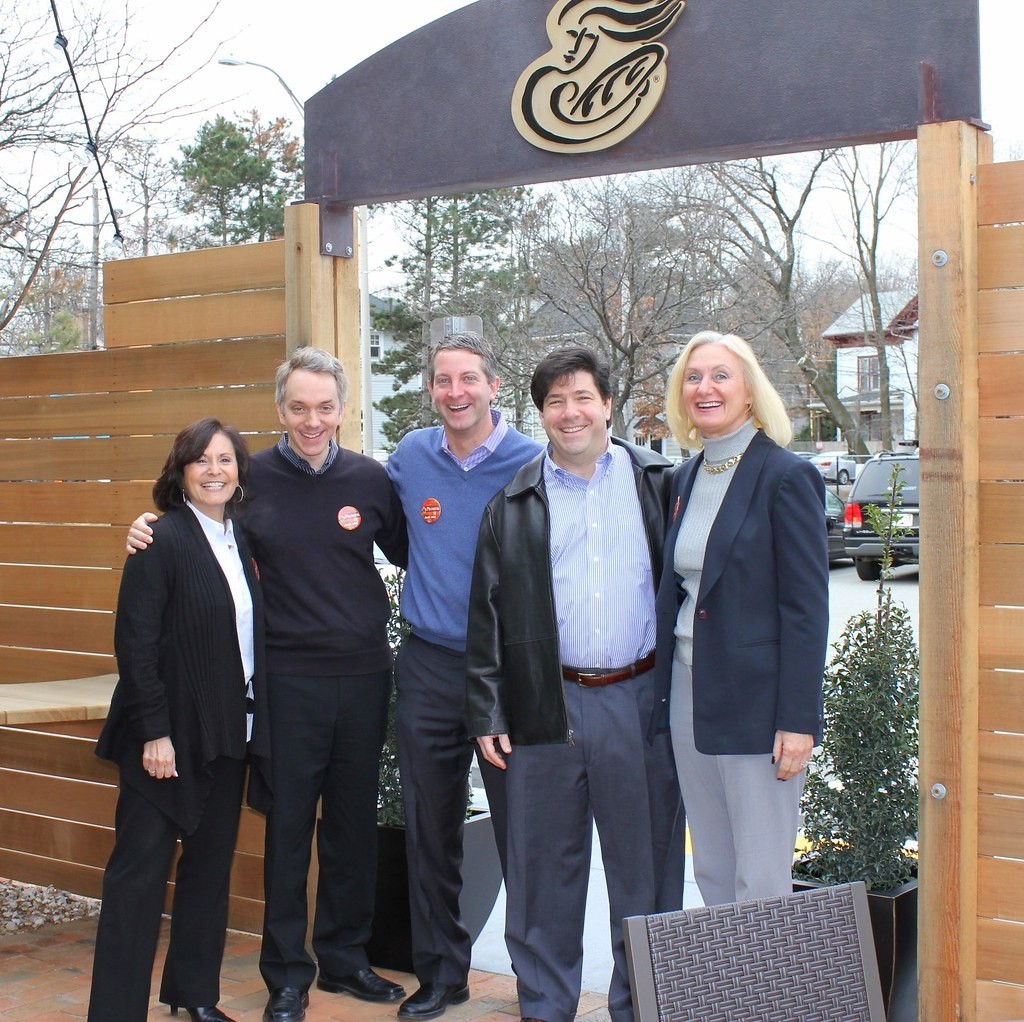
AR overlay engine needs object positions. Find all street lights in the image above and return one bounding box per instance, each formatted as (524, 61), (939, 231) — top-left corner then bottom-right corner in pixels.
(91, 189), (123, 352)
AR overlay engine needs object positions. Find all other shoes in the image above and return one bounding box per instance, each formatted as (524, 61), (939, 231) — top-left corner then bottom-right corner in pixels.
(520, 1018), (547, 1022)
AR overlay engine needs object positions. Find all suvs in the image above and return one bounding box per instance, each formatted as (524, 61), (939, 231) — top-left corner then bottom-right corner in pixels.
(842, 448), (920, 580)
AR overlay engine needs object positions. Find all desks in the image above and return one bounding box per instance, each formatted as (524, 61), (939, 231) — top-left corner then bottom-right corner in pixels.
(0, 673), (120, 725)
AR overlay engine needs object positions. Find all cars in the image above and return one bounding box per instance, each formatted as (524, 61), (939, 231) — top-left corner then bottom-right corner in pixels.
(824, 491), (854, 567)
(793, 452), (817, 461)
(808, 451), (857, 485)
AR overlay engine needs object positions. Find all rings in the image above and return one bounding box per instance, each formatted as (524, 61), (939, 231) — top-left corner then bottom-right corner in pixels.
(800, 762), (808, 768)
(148, 769), (156, 774)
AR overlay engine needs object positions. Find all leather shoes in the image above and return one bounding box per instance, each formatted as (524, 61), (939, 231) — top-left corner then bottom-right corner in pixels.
(317, 967), (406, 1001)
(263, 987), (309, 1022)
(397, 984), (470, 1019)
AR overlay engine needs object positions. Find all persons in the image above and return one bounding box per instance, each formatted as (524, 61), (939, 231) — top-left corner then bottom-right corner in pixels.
(84, 419), (260, 1022)
(652, 330), (830, 908)
(462, 345), (685, 1022)
(126, 345), (410, 1022)
(381, 333), (544, 1020)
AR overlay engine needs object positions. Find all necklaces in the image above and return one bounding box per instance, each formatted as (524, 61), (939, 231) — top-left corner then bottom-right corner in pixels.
(702, 452), (744, 473)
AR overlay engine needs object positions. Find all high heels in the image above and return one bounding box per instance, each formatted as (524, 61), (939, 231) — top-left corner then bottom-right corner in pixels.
(171, 1001), (236, 1022)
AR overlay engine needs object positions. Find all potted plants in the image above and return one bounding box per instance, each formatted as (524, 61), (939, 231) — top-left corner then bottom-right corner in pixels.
(792, 462), (919, 1022)
(316, 566), (504, 973)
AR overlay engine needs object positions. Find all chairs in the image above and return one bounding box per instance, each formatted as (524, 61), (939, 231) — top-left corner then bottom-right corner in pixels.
(624, 881), (887, 1022)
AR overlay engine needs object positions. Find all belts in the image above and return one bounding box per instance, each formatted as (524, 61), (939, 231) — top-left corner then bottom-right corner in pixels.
(563, 650), (656, 687)
